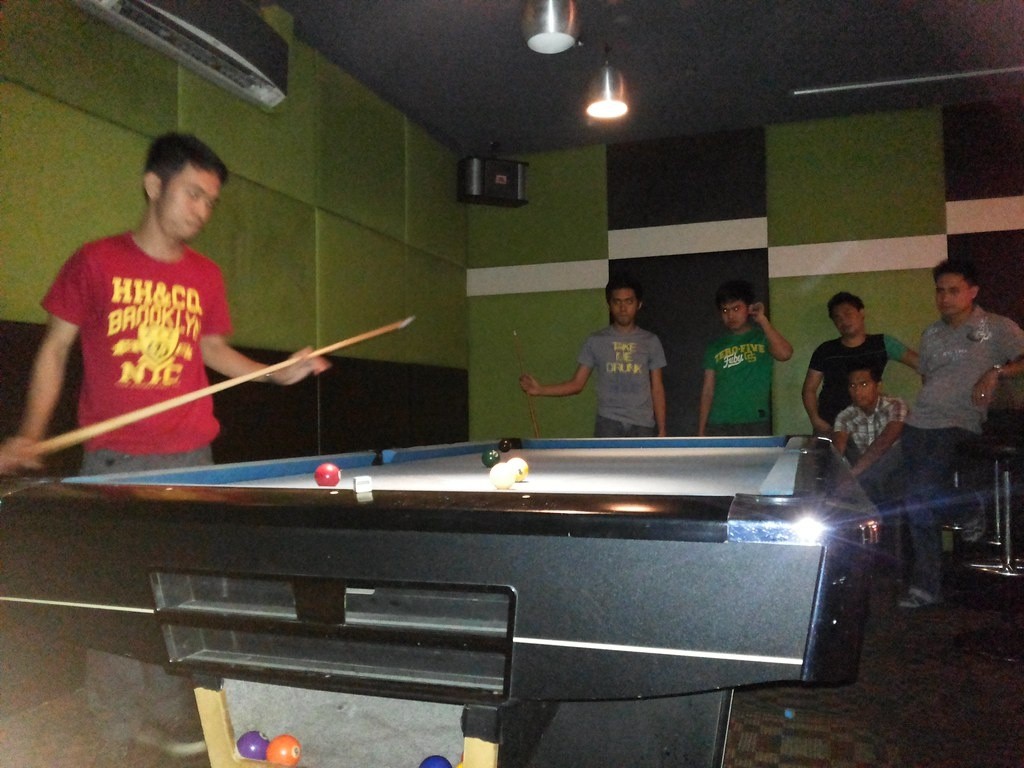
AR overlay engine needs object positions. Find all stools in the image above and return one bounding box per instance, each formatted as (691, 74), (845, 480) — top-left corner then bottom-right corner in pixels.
(953, 434), (1024, 577)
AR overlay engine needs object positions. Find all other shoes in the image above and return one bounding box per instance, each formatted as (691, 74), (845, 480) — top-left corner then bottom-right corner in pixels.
(898, 595), (929, 607)
(960, 492), (985, 542)
(136, 720), (206, 755)
(909, 586), (946, 604)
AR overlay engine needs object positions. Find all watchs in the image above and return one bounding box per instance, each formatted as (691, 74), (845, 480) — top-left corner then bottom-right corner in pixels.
(993, 364), (1003, 381)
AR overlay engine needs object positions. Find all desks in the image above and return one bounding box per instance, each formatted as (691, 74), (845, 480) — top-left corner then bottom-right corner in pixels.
(0, 436), (879, 767)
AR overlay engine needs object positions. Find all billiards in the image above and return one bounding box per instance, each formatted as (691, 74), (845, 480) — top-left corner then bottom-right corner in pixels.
(490, 462), (516, 489)
(507, 457), (528, 483)
(499, 439), (512, 452)
(314, 462), (342, 487)
(482, 448), (500, 468)
(418, 754), (452, 768)
(266, 734), (302, 766)
(236, 730), (270, 760)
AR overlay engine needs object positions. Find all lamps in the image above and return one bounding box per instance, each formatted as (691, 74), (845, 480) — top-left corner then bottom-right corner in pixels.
(520, 0), (580, 55)
(582, 44), (629, 120)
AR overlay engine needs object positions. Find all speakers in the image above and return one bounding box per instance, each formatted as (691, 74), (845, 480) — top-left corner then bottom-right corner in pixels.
(457, 157), (529, 207)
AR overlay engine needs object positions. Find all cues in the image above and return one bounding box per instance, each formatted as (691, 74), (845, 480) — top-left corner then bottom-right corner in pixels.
(27, 316), (418, 456)
(512, 329), (540, 439)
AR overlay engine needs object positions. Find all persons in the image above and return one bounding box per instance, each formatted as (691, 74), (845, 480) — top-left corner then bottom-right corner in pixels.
(800, 291), (920, 439)
(518, 273), (668, 437)
(1, 131), (331, 759)
(832, 358), (921, 608)
(698, 278), (794, 437)
(900, 260), (1024, 608)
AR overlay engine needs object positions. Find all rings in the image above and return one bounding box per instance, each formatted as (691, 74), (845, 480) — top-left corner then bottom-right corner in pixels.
(980, 393), (984, 399)
